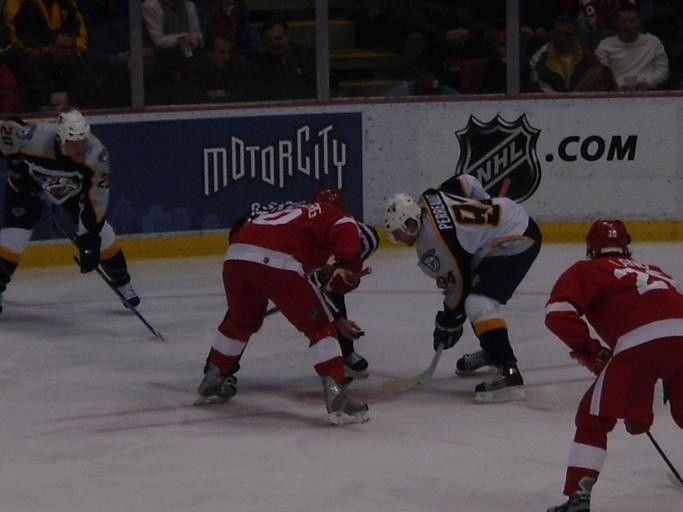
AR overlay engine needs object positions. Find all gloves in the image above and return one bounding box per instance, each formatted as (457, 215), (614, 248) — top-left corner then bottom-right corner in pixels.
(432, 310), (468, 351)
(590, 347), (612, 375)
(77, 230), (102, 276)
(325, 262), (362, 294)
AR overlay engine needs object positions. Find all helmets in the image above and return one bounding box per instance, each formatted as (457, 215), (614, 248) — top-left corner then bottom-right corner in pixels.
(54, 109), (89, 144)
(383, 193), (422, 237)
(360, 217), (380, 263)
(311, 185), (349, 212)
(584, 219), (631, 256)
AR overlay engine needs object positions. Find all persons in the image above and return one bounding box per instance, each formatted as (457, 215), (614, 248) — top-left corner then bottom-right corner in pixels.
(0, 107), (141, 311)
(545, 218), (683, 512)
(308, 220), (370, 387)
(384, 174), (542, 402)
(195, 187), (371, 427)
(386, 2), (666, 99)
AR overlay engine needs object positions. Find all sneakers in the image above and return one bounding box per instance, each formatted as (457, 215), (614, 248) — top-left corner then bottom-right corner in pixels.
(337, 343), (367, 370)
(321, 376), (369, 416)
(455, 345), (495, 370)
(115, 280), (140, 309)
(475, 361), (525, 394)
(196, 362), (237, 400)
(543, 491), (593, 512)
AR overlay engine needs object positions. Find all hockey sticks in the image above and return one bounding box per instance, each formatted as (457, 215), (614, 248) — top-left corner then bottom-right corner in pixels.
(382, 176), (513, 395)
(34, 190), (165, 344)
